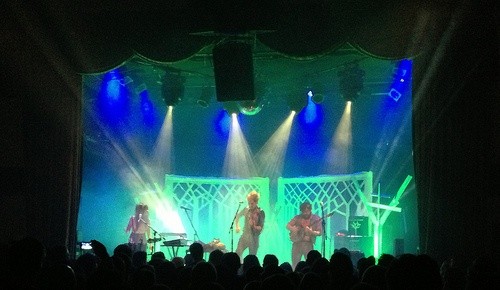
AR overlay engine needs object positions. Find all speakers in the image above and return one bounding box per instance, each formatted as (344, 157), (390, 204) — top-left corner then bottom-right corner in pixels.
(213, 42), (256, 102)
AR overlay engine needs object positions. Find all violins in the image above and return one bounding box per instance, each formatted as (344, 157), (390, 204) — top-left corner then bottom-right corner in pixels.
(245, 210), (260, 235)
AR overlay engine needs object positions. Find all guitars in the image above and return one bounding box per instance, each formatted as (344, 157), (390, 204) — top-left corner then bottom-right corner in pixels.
(290, 210), (335, 243)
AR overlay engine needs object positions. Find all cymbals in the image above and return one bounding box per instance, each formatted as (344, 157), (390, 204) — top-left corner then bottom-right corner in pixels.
(147, 238), (161, 243)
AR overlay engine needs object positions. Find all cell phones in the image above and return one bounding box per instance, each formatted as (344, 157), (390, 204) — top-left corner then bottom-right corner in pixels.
(80, 241), (93, 250)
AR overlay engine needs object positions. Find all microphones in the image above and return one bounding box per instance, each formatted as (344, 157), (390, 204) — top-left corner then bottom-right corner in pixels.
(239, 201), (244, 203)
(180, 206), (191, 210)
(138, 214), (142, 221)
(320, 202), (324, 207)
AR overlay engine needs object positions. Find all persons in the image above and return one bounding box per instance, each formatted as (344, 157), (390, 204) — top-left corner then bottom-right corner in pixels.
(235, 190), (266, 257)
(0, 236), (500, 290)
(124, 203), (153, 253)
(286, 202), (322, 271)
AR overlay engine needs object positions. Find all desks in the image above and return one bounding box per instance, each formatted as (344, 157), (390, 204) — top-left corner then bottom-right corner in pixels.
(160, 244), (188, 259)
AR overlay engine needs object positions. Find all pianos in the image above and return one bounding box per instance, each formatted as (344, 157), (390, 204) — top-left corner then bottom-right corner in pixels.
(161, 239), (193, 259)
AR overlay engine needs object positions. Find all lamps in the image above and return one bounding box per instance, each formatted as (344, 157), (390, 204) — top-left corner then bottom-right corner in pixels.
(287, 94), (309, 114)
(197, 88), (211, 107)
(388, 58), (413, 101)
(310, 92), (327, 104)
(225, 103), (240, 116)
(159, 65), (185, 108)
(127, 74), (146, 95)
(338, 61), (364, 103)
